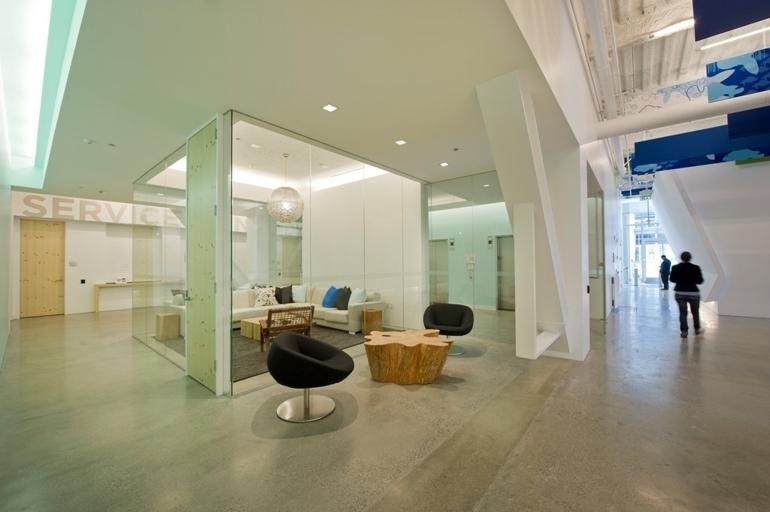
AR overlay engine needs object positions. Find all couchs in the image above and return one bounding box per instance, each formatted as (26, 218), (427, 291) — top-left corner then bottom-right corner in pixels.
(232, 288), (386, 335)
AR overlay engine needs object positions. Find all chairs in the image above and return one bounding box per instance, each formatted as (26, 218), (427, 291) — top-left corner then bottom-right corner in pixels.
(261, 305), (314, 351)
(423, 302), (474, 355)
(268, 334), (355, 424)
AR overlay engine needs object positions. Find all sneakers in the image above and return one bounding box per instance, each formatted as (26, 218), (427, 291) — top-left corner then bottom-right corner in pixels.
(696, 328), (705, 335)
(681, 332), (688, 337)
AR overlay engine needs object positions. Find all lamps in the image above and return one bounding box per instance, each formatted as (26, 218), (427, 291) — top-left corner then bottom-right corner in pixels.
(269, 152), (303, 223)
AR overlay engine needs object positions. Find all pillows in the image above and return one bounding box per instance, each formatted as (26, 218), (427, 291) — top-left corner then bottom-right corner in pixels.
(253, 284), (367, 311)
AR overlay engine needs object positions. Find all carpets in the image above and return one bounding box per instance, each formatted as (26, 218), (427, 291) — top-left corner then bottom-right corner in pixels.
(151, 329), (370, 384)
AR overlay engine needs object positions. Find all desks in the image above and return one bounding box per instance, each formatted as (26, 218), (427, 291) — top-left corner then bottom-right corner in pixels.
(95, 280), (185, 322)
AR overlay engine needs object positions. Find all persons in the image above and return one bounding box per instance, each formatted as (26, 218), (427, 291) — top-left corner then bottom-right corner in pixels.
(669, 251), (704, 337)
(658, 254), (672, 291)
